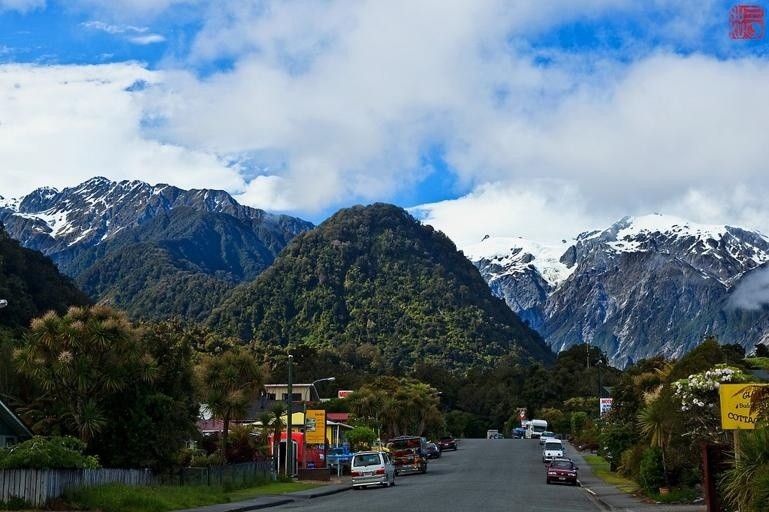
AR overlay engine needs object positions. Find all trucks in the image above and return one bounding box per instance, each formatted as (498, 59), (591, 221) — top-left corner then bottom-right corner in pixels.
(388, 435), (428, 474)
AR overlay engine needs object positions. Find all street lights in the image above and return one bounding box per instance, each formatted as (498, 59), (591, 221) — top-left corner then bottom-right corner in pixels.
(301, 376), (335, 466)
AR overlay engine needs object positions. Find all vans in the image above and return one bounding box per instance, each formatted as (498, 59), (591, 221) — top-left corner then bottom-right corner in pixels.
(526, 417), (548, 438)
(542, 439), (565, 462)
(351, 450), (396, 487)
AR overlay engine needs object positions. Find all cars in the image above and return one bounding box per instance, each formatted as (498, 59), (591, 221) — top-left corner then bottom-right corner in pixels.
(546, 456), (580, 484)
(427, 442), (440, 457)
(512, 427), (527, 439)
(438, 435), (457, 450)
(540, 431), (555, 444)
